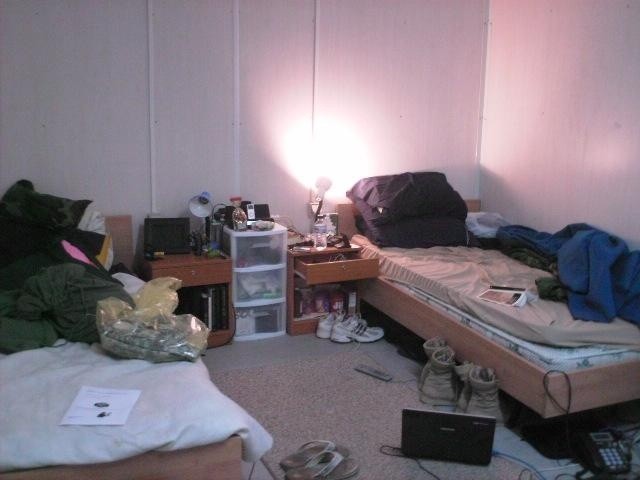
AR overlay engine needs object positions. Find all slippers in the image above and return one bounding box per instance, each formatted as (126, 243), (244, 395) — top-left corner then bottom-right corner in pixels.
(286, 451), (360, 480)
(281, 439), (349, 470)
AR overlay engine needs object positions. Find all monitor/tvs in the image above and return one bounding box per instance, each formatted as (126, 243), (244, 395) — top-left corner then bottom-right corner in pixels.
(144, 217), (191, 254)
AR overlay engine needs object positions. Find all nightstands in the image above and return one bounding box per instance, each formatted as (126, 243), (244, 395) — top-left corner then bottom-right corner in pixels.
(142, 250), (236, 349)
(286, 236), (379, 336)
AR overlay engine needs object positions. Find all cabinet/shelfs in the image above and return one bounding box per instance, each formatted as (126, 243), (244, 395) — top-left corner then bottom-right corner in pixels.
(224, 222), (287, 341)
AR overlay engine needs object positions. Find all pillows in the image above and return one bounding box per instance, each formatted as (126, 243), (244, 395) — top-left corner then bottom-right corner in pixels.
(347, 171), (467, 247)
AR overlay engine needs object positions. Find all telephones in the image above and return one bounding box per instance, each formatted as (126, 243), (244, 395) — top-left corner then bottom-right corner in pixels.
(568, 427), (631, 476)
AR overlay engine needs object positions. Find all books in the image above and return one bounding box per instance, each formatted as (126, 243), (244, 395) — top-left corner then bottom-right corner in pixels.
(476, 285), (539, 308)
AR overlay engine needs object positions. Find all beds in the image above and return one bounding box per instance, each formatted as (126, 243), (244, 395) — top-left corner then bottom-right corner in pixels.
(0, 216), (243, 480)
(337, 203), (639, 458)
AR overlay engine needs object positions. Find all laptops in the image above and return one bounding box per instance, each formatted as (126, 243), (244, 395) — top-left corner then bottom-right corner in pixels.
(401, 408), (496, 466)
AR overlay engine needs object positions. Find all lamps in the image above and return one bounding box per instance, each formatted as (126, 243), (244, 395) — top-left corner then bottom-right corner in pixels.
(313, 177), (332, 221)
(188, 192), (219, 253)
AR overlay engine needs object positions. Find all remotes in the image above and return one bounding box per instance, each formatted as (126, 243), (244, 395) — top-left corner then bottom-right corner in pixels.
(356, 364), (392, 382)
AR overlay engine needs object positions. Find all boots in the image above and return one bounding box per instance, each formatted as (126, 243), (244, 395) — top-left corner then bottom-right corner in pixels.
(419, 336), (526, 422)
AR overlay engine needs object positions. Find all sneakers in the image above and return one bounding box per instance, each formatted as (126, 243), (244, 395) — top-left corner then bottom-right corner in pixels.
(316, 312), (367, 339)
(331, 316), (384, 342)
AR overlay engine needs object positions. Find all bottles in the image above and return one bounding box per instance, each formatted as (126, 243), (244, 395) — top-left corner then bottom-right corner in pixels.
(313, 214), (327, 251)
